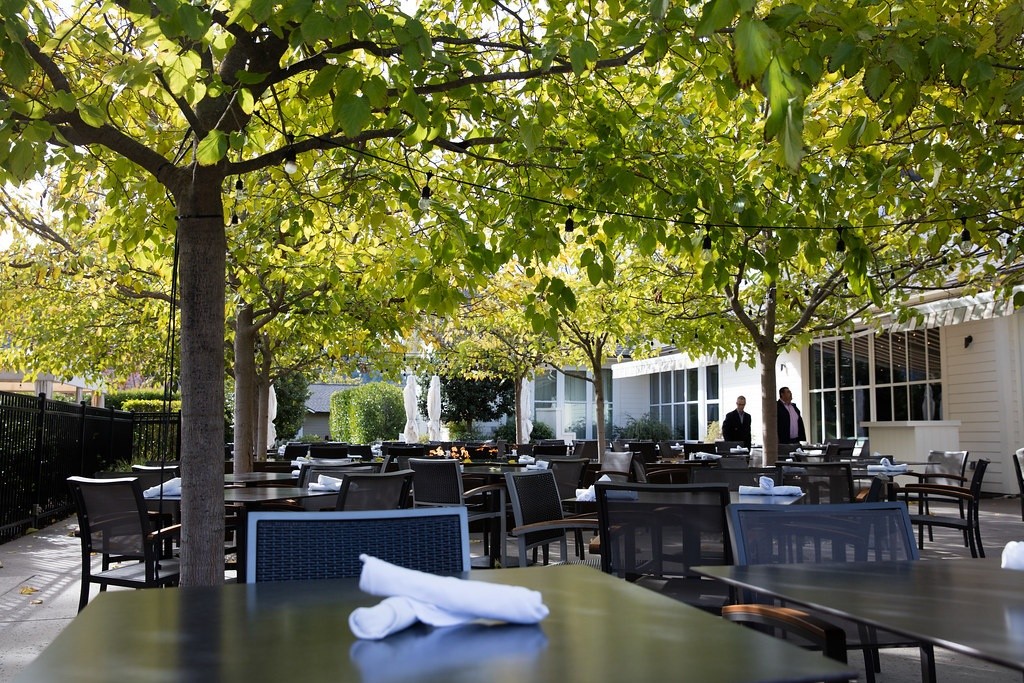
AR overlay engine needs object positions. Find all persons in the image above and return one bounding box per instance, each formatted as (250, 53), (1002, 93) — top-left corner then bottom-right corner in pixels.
(722, 396), (751, 464)
(776, 387), (807, 443)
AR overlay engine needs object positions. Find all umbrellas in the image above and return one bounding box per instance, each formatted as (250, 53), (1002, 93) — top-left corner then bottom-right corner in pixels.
(267, 385), (277, 449)
(403, 376), (419, 443)
(426, 376), (441, 441)
(516, 376), (533, 444)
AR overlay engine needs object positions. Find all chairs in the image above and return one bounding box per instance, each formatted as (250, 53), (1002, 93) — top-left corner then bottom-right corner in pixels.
(67, 439), (1024, 683)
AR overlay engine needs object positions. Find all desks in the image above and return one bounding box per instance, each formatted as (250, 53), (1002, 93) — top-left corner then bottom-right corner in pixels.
(559, 491), (808, 601)
(222, 470), (300, 488)
(775, 468), (913, 560)
(144, 485), (373, 584)
(460, 466), (537, 567)
(587, 462), (602, 469)
(20, 561), (860, 683)
(650, 462), (718, 483)
(692, 557), (1024, 673)
(856, 460), (941, 465)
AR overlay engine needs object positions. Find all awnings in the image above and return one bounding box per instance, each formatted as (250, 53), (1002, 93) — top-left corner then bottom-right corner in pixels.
(611, 346), (729, 379)
(888, 285), (1024, 333)
